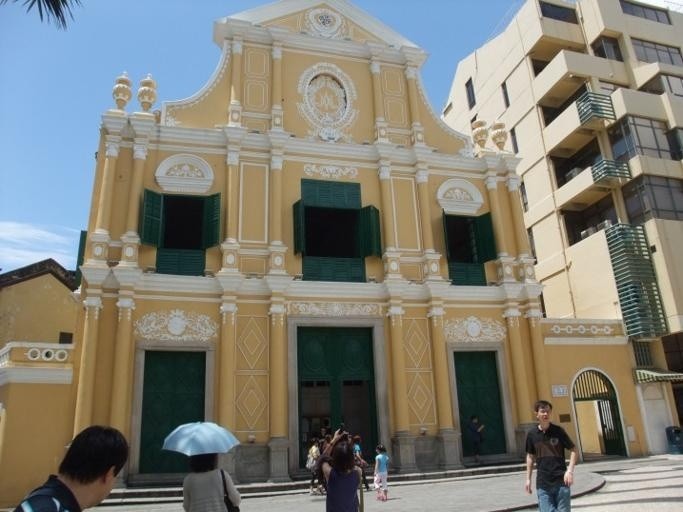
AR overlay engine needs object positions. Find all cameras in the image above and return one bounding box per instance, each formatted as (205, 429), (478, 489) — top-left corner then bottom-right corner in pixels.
(339, 423), (349, 441)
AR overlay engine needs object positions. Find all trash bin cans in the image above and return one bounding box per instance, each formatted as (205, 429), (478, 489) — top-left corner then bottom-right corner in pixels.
(665, 426), (683, 454)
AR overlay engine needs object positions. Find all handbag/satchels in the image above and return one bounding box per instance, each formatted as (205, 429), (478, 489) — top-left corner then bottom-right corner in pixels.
(223, 495), (241, 511)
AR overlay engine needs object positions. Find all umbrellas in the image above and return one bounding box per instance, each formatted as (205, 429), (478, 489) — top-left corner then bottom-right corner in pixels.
(160, 420), (240, 459)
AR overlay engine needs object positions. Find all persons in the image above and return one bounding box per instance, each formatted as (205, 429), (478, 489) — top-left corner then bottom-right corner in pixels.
(12, 424), (128, 511)
(304, 427), (370, 495)
(523, 400), (578, 512)
(372, 444), (390, 501)
(467, 415), (486, 464)
(315, 428), (363, 511)
(182, 453), (241, 512)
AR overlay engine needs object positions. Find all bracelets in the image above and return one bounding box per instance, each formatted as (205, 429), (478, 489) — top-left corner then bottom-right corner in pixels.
(568, 470), (573, 474)
